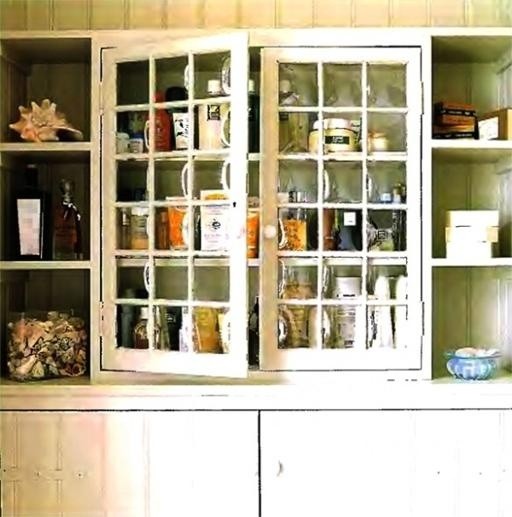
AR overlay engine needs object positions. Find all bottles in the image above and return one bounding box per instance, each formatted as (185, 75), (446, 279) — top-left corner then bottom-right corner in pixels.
(12, 163), (82, 261)
(289, 190), (308, 250)
(149, 79), (302, 152)
(134, 307), (161, 350)
(121, 208), (169, 250)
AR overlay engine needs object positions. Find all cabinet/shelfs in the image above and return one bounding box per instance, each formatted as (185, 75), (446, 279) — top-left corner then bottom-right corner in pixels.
(1, 24), (512, 382)
(1, 408), (509, 517)
